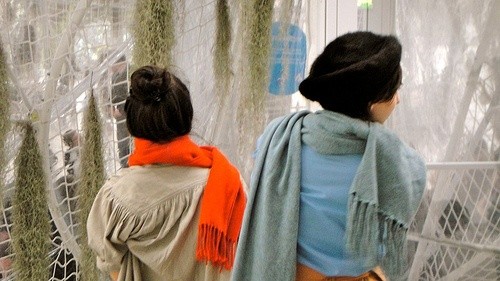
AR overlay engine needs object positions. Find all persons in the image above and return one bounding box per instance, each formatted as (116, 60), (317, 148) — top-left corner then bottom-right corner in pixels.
(84, 65), (250, 280)
(251, 30), (428, 281)
